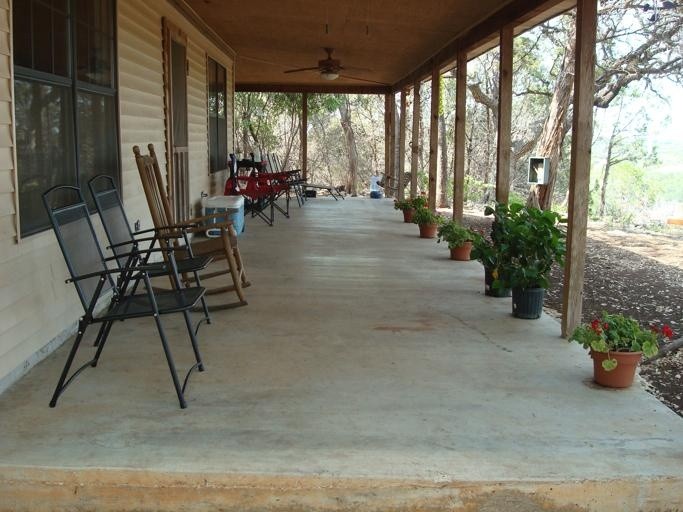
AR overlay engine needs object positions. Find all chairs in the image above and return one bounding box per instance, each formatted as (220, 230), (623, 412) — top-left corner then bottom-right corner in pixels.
(87, 174), (213, 350)
(226, 153), (345, 228)
(132, 143), (251, 311)
(41, 184), (207, 409)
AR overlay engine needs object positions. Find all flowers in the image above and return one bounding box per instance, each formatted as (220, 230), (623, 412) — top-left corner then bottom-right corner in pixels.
(567, 311), (674, 357)
(393, 192), (430, 212)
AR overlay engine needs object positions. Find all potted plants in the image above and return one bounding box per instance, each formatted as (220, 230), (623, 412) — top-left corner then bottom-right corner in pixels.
(412, 207), (445, 238)
(500, 203), (567, 319)
(436, 219), (485, 260)
(471, 200), (525, 297)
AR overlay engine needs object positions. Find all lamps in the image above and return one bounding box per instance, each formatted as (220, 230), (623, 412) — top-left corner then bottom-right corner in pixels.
(320, 69), (340, 80)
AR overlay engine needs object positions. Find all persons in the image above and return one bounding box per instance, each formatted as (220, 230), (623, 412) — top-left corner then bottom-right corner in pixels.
(369, 169), (384, 199)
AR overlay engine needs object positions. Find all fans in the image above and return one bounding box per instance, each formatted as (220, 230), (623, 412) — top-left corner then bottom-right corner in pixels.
(283, 47), (376, 74)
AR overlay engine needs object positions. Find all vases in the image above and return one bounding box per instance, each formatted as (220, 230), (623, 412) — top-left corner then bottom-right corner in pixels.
(403, 207), (418, 223)
(589, 345), (643, 388)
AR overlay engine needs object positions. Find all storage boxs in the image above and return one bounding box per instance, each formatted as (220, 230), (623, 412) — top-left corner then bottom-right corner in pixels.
(201, 196), (244, 238)
